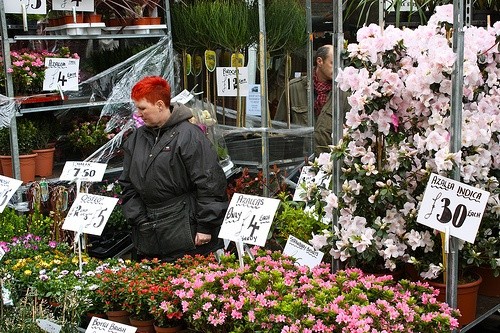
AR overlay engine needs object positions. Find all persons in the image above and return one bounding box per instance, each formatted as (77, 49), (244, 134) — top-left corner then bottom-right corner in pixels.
(121, 76), (227, 261)
(273, 45), (353, 160)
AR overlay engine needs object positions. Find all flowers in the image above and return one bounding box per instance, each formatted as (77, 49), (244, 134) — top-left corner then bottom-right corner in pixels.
(0, 4), (500, 333)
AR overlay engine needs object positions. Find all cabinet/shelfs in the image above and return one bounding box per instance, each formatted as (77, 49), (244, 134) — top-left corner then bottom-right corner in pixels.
(0, 0), (174, 212)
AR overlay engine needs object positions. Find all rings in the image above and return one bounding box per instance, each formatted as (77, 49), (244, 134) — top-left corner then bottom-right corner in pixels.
(202, 243), (204, 244)
(205, 242), (208, 243)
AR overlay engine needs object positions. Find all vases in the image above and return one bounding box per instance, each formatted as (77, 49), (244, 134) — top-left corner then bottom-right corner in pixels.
(475, 265), (500, 297)
(110, 19), (119, 27)
(85, 13), (102, 22)
(120, 18), (132, 26)
(154, 320), (181, 333)
(77, 14), (84, 23)
(48, 18), (53, 27)
(32, 144), (55, 177)
(418, 272), (482, 327)
(58, 16), (64, 25)
(53, 19), (59, 26)
(106, 310), (129, 323)
(0, 152), (39, 185)
(129, 314), (154, 333)
(133, 17), (150, 25)
(64, 15), (73, 23)
(149, 17), (161, 24)
